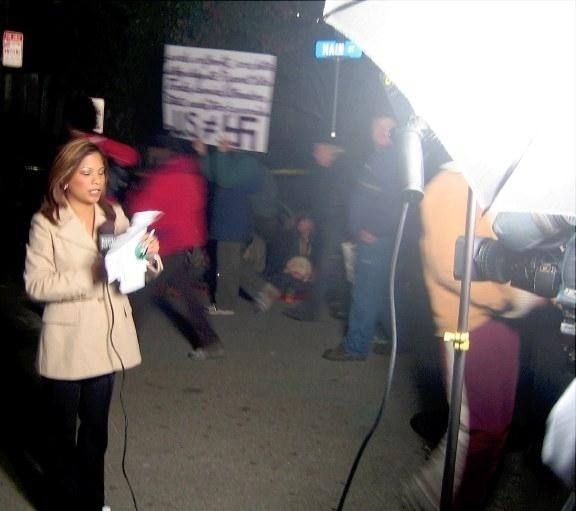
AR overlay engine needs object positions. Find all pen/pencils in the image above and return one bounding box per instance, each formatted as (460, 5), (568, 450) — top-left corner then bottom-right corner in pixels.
(142, 229), (156, 256)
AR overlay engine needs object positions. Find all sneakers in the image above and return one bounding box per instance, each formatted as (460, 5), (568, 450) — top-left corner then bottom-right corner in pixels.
(330, 300), (350, 319)
(207, 304), (233, 316)
(284, 305), (315, 320)
(374, 339), (406, 355)
(191, 346), (223, 361)
(324, 346), (366, 362)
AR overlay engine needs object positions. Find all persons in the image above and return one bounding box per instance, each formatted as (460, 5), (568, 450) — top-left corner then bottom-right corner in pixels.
(276, 125), (360, 323)
(23, 137), (163, 511)
(324, 115), (414, 361)
(398, 166), (555, 511)
(123, 130), (229, 362)
(192, 134), (281, 316)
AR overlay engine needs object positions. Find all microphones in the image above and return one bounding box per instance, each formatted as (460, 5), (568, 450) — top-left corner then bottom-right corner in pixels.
(97, 220), (116, 256)
